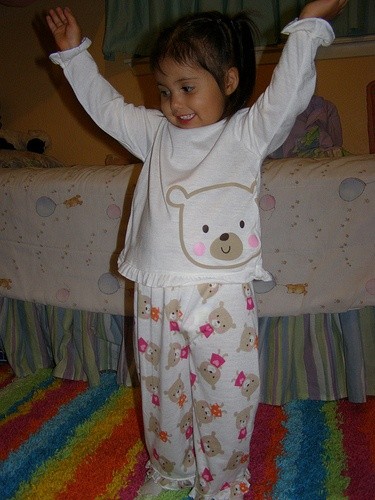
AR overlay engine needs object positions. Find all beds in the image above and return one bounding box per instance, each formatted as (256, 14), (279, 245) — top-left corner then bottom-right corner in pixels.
(0, 151), (375, 407)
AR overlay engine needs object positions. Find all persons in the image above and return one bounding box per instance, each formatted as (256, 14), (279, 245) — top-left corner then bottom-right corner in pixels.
(43, 0), (348, 500)
